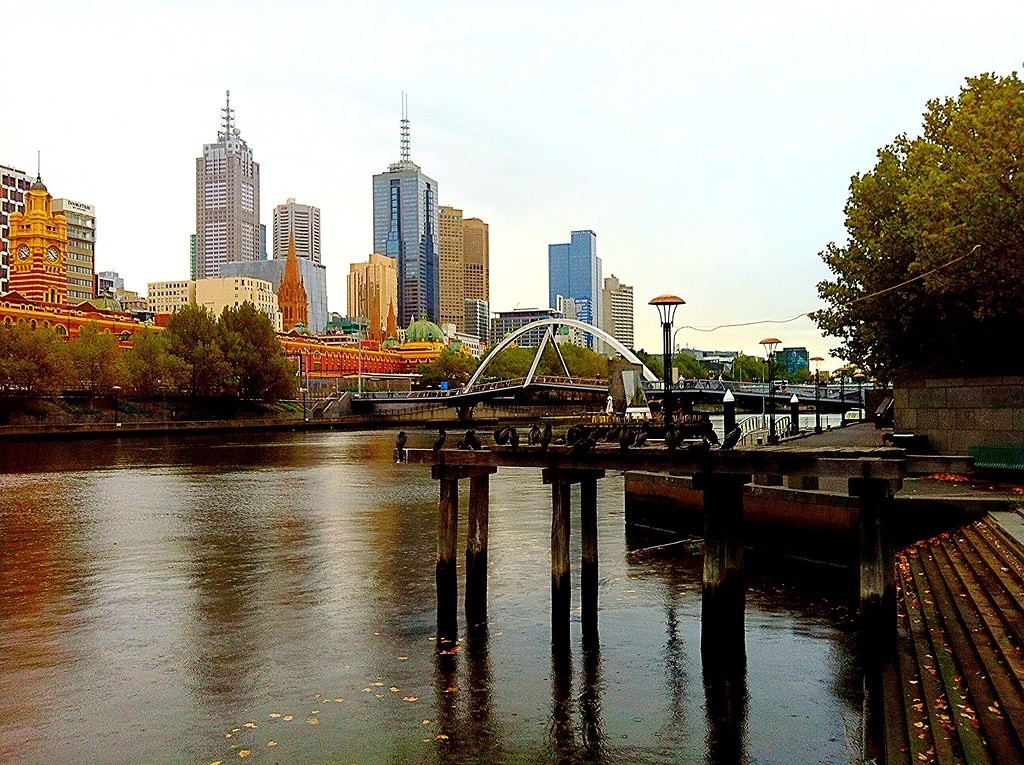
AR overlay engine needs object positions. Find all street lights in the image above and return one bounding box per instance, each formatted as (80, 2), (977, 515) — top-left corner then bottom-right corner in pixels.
(300, 389), (308, 421)
(809, 357), (824, 432)
(759, 338), (782, 442)
(648, 294), (686, 445)
(854, 374), (865, 422)
(112, 386), (121, 424)
(837, 368), (849, 426)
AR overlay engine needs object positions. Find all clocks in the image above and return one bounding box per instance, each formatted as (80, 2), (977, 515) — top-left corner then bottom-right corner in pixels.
(17, 245), (31, 263)
(45, 245), (60, 264)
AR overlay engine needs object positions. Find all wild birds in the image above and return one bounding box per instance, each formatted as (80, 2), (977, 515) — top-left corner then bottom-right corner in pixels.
(393, 420), (741, 463)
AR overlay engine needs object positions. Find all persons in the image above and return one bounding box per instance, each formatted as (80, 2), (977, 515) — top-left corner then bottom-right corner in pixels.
(596, 372), (602, 385)
(704, 373), (711, 389)
(387, 388), (395, 398)
(714, 374), (726, 391)
(363, 389), (376, 398)
